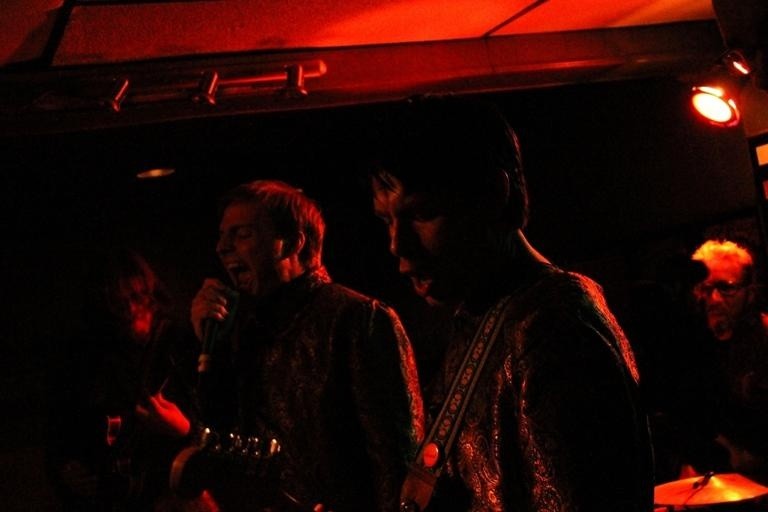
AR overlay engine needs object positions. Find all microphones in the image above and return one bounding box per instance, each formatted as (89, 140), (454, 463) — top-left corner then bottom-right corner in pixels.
(197, 289), (234, 373)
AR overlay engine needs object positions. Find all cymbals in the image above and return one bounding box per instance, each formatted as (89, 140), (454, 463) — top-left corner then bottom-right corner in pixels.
(654, 475), (768, 506)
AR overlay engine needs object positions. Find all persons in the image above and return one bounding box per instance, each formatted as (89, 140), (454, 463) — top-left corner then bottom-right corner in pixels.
(360, 90), (658, 510)
(132, 179), (426, 510)
(99, 249), (219, 512)
(680, 233), (767, 484)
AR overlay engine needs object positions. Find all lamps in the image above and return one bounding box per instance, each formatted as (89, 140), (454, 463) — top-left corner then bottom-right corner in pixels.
(687, 46), (758, 128)
